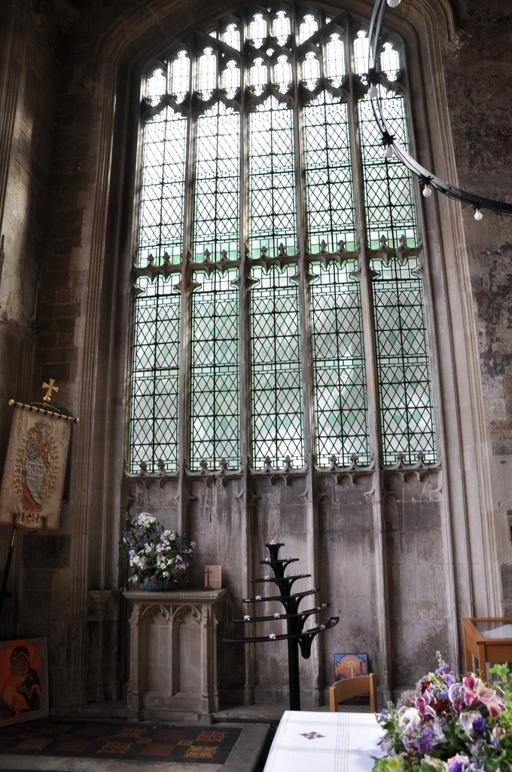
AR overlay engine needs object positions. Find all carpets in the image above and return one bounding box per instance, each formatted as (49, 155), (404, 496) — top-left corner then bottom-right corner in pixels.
(0, 718), (243, 764)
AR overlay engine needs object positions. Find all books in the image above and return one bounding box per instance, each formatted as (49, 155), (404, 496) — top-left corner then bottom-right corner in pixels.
(204, 564), (222, 589)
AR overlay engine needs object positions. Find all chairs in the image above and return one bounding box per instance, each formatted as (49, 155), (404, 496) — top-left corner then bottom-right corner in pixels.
(329, 672), (376, 712)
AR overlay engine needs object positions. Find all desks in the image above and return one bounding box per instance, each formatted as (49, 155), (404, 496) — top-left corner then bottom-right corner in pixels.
(263, 710), (396, 772)
(461, 617), (512, 686)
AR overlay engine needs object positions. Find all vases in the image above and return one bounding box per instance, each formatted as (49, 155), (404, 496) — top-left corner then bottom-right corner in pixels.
(144, 580), (171, 590)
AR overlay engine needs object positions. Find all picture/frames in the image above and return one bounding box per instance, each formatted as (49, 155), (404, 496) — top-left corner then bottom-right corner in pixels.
(334, 653), (368, 682)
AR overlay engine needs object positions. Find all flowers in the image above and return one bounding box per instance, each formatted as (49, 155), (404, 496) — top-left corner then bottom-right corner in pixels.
(369, 649), (512, 772)
(117, 512), (197, 590)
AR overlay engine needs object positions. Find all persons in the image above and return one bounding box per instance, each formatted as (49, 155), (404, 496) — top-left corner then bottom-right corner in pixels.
(19, 427), (44, 515)
(3, 646), (43, 716)
